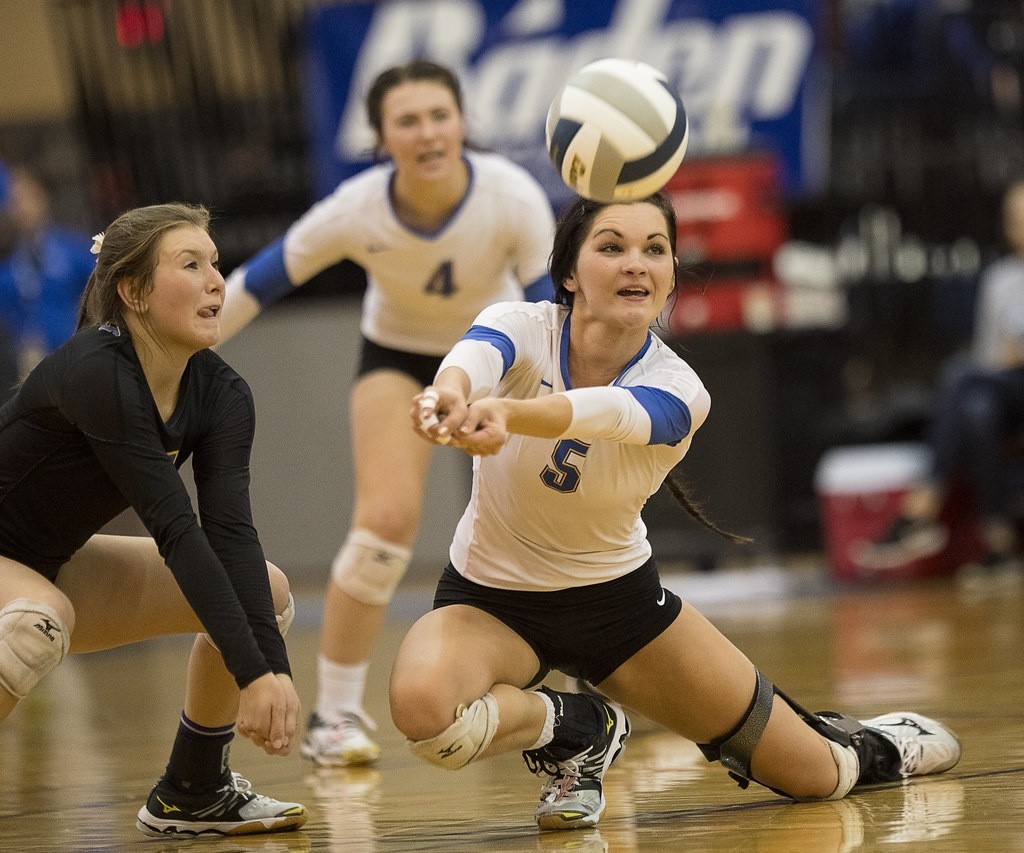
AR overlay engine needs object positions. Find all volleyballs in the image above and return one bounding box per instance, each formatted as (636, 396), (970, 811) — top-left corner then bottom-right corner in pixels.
(545, 57), (689, 203)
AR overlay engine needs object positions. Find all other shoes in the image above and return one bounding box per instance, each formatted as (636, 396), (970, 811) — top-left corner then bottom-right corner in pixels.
(849, 511), (951, 571)
(305, 698), (382, 768)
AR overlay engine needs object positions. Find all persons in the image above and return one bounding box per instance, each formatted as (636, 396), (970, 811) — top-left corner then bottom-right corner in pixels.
(944, 178), (1024, 568)
(207, 57), (556, 767)
(0, 200), (310, 838)
(0, 161), (98, 397)
(390, 193), (960, 829)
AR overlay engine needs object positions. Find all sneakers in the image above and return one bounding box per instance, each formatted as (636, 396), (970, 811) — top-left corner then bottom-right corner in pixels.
(137, 771), (310, 841)
(812, 709), (963, 779)
(525, 685), (631, 833)
